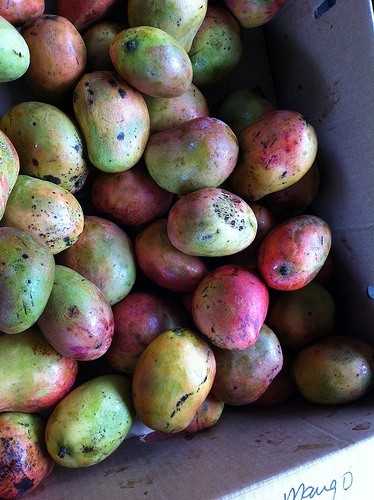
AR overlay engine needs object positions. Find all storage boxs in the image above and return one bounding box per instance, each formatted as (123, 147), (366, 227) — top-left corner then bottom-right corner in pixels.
(0, 0), (374, 500)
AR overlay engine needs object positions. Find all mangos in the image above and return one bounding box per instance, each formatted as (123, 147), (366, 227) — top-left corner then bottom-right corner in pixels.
(0, 0), (374, 500)
